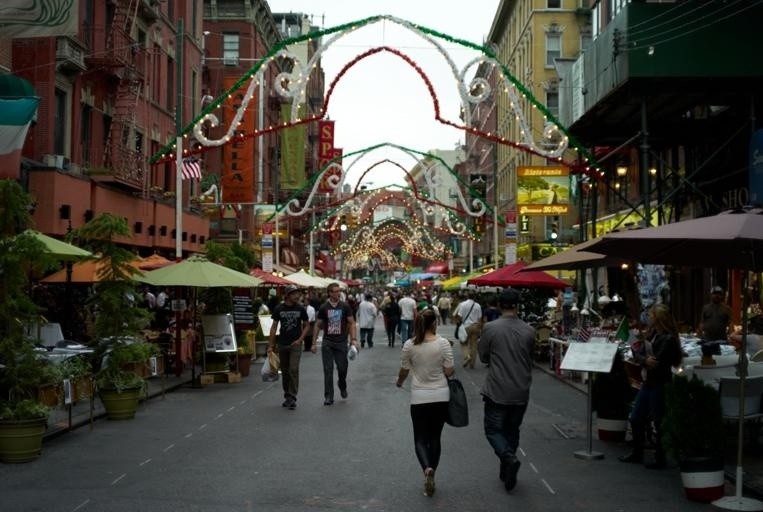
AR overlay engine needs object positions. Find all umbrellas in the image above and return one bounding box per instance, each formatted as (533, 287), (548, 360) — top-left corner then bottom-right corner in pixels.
(132, 255), (265, 386)
(42, 252), (151, 284)
(468, 261), (574, 291)
(133, 253), (175, 268)
(578, 206), (763, 502)
(513, 226), (676, 311)
(2, 227), (101, 299)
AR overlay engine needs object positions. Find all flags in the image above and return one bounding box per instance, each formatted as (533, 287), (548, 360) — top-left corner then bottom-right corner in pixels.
(0, 97), (41, 180)
(614, 316), (629, 342)
(173, 154), (202, 181)
(579, 328), (589, 342)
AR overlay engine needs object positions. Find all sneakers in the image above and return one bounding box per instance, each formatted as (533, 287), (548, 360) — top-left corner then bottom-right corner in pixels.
(470, 364), (474, 368)
(424, 466), (435, 498)
(463, 354), (472, 368)
(499, 461), (505, 482)
(341, 390), (347, 398)
(324, 399), (334, 406)
(288, 400), (297, 410)
(282, 400), (288, 407)
(504, 457), (521, 491)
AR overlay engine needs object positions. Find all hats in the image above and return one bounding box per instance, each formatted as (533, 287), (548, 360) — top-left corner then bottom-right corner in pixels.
(284, 284), (300, 297)
(497, 288), (519, 308)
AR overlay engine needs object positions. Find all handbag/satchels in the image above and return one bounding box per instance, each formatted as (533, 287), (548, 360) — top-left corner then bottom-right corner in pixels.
(455, 321), (463, 339)
(457, 324), (469, 344)
(444, 378), (469, 428)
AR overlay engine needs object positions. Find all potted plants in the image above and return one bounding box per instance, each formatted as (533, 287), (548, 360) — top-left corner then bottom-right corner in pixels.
(228, 330), (255, 376)
(0, 178), (49, 464)
(65, 208), (158, 420)
(29, 359), (60, 405)
(120, 340), (164, 379)
(59, 354), (98, 403)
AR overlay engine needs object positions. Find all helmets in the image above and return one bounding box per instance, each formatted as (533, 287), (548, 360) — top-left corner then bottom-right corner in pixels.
(711, 286), (723, 294)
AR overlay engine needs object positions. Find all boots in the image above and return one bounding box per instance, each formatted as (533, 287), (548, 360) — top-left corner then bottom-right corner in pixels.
(616, 417), (646, 464)
(644, 418), (668, 469)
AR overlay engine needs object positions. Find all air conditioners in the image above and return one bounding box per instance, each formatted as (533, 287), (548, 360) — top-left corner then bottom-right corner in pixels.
(224, 57), (239, 66)
(56, 155), (71, 169)
(44, 154), (56, 167)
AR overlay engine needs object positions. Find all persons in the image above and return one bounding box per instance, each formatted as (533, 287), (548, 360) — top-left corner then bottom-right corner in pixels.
(700, 286), (734, 341)
(546, 291), (566, 369)
(397, 309), (455, 497)
(476, 289), (536, 490)
(267, 284), (310, 410)
(311, 282), (359, 405)
(250, 267), (502, 369)
(618, 305), (682, 462)
(139, 286), (157, 330)
(157, 287), (171, 330)
